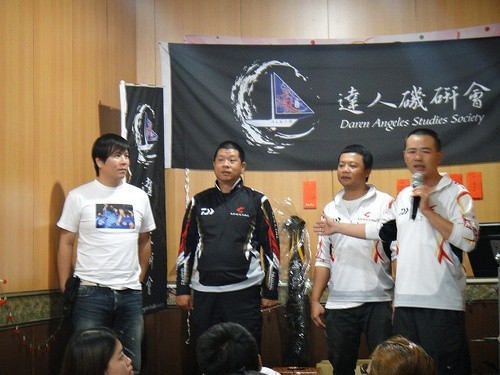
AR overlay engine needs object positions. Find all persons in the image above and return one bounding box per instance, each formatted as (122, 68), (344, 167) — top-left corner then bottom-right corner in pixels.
(175, 142), (281, 375)
(311, 144), (398, 375)
(55, 134), (156, 375)
(195, 322), (266, 375)
(62, 326), (135, 375)
(313, 128), (480, 375)
(316, 337), (435, 375)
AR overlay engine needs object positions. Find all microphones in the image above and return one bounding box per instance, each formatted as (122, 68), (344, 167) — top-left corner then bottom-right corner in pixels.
(412, 173), (424, 220)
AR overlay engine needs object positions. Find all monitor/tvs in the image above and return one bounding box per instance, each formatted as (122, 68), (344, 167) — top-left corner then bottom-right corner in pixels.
(466, 222), (500, 278)
